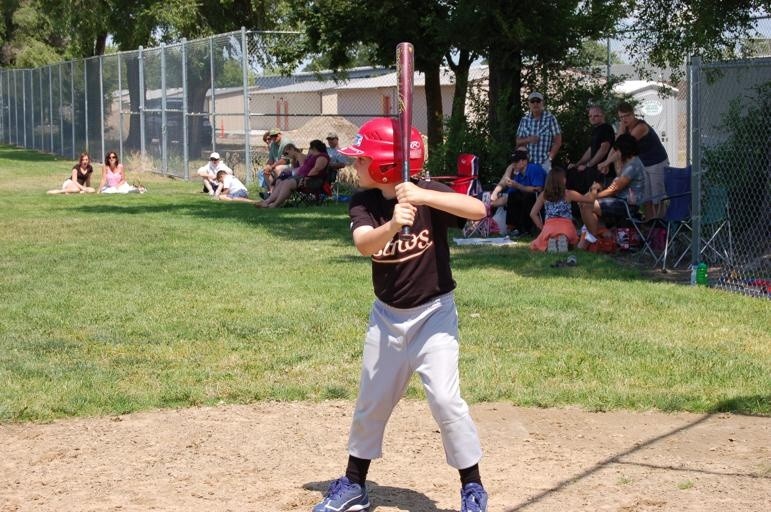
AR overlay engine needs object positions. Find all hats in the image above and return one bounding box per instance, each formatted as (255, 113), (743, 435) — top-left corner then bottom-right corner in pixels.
(505, 153), (528, 165)
(210, 153), (220, 160)
(528, 92), (543, 100)
(326, 132), (337, 140)
(269, 129), (281, 137)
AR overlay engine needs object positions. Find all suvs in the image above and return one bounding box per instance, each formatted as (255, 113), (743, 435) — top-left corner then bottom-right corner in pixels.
(148, 100), (212, 147)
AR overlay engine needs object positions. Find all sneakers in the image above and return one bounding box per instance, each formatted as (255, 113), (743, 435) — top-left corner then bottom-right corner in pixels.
(461, 482), (486, 512)
(548, 234), (568, 252)
(551, 255), (576, 267)
(313, 477), (371, 512)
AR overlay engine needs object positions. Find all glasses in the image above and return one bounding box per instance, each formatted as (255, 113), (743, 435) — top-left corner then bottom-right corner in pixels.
(108, 156), (116, 159)
(530, 99), (541, 103)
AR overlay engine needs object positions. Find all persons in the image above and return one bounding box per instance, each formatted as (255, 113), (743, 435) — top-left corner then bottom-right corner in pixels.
(482, 91), (668, 255)
(197, 152), (233, 195)
(253, 126), (351, 209)
(46, 152), (96, 194)
(309, 118), (489, 512)
(97, 151), (146, 194)
(216, 170), (256, 202)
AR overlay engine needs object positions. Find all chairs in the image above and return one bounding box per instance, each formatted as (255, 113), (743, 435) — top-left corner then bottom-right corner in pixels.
(416, 154), (481, 227)
(494, 167), (732, 268)
(290, 159), (341, 208)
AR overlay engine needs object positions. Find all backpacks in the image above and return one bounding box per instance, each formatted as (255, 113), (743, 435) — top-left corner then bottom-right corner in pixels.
(564, 125), (607, 161)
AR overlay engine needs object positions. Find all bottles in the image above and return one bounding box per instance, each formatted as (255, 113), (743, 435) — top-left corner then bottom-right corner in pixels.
(691, 260), (699, 285)
(698, 261), (708, 285)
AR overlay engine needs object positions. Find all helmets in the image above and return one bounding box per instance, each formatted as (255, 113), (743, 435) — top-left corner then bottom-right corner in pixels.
(337, 118), (424, 184)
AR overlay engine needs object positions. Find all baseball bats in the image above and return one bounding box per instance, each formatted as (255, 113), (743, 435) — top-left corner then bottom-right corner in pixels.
(397, 42), (414, 242)
